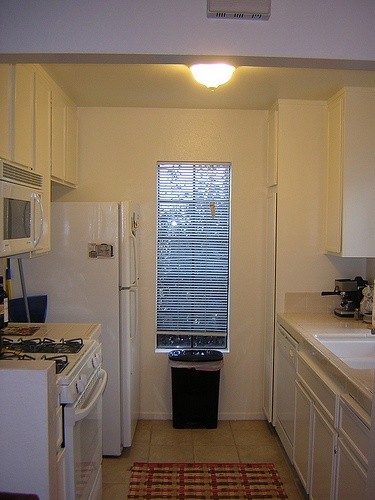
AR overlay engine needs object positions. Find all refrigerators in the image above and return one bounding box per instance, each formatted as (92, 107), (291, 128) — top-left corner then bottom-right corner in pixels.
(10, 200), (140, 455)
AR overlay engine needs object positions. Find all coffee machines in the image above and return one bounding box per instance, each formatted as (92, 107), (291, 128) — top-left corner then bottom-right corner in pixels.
(334, 276), (369, 317)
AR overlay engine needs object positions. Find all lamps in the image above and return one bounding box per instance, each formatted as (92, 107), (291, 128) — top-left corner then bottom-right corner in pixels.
(185, 62), (239, 93)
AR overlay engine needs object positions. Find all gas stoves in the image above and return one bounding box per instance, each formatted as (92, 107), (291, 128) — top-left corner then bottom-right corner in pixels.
(0, 335), (103, 405)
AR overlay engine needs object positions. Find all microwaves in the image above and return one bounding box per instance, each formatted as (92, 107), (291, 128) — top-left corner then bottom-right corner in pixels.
(0, 179), (45, 258)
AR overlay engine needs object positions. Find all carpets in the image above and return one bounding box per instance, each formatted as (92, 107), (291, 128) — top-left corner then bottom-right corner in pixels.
(128, 462), (289, 500)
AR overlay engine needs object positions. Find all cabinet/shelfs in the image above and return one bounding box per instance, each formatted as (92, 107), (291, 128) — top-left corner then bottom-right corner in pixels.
(262, 99), (367, 429)
(326, 86), (375, 258)
(293, 343), (344, 500)
(0, 63), (15, 161)
(11, 63), (51, 258)
(51, 80), (79, 189)
(334, 386), (375, 500)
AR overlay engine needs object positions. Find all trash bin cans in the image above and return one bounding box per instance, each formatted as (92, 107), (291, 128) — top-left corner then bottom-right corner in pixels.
(167, 350), (224, 430)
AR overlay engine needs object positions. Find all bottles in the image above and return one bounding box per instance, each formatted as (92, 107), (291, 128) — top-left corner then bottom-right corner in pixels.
(0, 276), (9, 327)
(354, 308), (360, 320)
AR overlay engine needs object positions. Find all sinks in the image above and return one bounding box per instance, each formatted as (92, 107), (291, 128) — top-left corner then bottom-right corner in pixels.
(312, 333), (375, 370)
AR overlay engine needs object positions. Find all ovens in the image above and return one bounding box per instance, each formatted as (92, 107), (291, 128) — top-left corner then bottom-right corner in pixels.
(58, 369), (109, 500)
